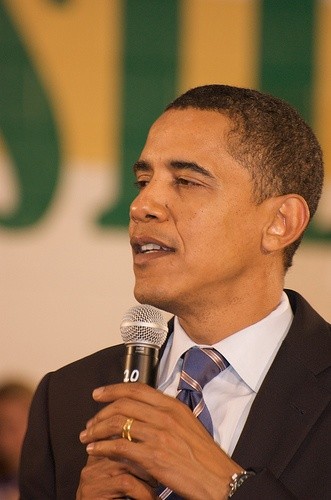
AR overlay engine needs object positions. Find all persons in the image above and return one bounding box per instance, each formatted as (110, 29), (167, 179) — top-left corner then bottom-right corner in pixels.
(17, 85), (331, 500)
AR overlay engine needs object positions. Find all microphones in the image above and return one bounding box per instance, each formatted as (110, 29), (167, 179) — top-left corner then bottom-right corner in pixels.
(119, 304), (169, 391)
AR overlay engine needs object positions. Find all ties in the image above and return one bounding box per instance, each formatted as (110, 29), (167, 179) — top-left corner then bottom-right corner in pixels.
(157, 345), (231, 500)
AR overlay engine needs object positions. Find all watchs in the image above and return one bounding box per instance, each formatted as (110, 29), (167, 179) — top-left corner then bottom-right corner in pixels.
(228, 471), (255, 497)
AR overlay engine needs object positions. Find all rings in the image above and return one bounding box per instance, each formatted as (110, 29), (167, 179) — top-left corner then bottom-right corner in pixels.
(122, 418), (133, 441)
